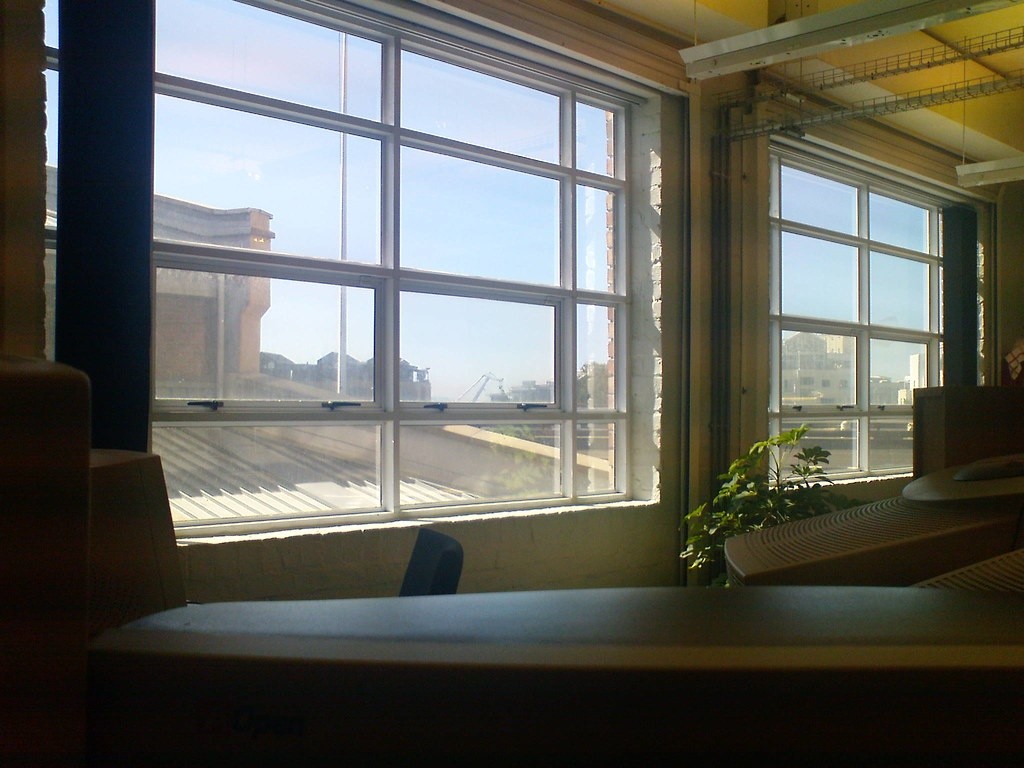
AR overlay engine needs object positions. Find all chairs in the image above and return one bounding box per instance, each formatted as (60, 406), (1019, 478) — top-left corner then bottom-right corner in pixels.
(398, 528), (464, 597)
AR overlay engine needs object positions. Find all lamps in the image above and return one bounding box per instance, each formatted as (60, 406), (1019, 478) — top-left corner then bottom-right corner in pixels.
(955, 156), (1024, 188)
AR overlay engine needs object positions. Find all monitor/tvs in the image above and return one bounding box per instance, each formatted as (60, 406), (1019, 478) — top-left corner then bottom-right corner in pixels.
(90, 449), (188, 646)
